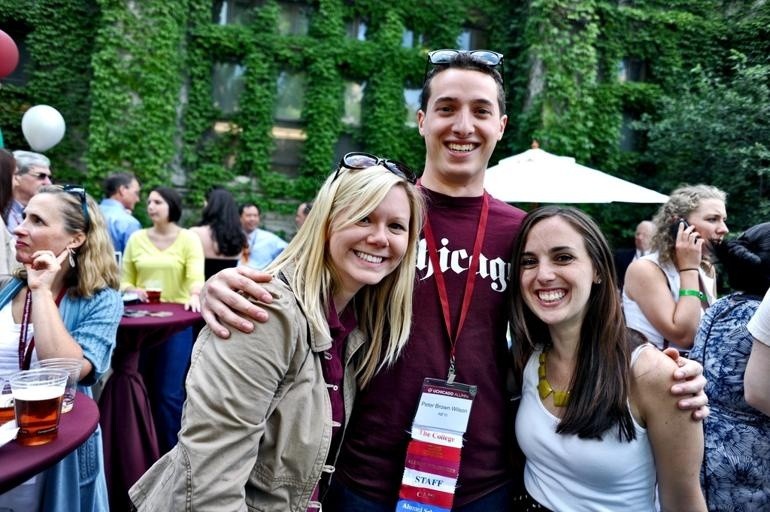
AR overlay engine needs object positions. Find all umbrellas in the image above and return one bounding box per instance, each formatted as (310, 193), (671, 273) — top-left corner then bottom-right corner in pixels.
(484, 140), (671, 209)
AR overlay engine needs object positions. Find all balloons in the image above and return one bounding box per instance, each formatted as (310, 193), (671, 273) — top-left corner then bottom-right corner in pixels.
(22, 105), (66, 152)
(0, 30), (19, 78)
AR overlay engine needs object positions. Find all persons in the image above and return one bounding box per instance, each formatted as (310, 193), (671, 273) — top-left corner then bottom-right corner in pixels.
(98, 171), (206, 452)
(511, 204), (709, 512)
(128, 153), (427, 512)
(197, 49), (711, 512)
(1, 149), (124, 511)
(187, 185), (313, 281)
(621, 183), (768, 510)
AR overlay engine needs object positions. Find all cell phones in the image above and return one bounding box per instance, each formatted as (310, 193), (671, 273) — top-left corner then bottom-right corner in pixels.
(669, 219), (695, 241)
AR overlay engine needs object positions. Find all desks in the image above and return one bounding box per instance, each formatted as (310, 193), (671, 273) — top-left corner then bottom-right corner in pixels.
(0, 376), (100, 512)
(95, 293), (210, 511)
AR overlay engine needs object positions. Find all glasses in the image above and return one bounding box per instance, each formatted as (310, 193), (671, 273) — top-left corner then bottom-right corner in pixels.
(63, 184), (89, 232)
(424, 48), (505, 91)
(27, 171), (52, 181)
(332, 151), (417, 185)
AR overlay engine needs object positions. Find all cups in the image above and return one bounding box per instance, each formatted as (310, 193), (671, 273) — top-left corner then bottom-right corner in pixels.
(9, 370), (68, 435)
(144, 281), (163, 303)
(31, 358), (84, 412)
